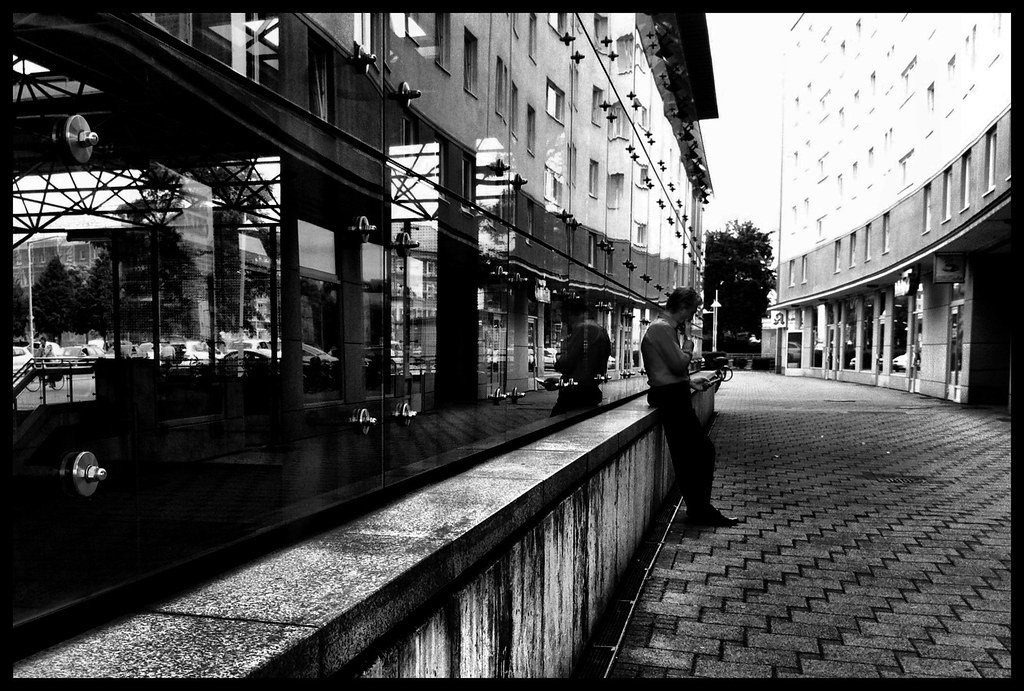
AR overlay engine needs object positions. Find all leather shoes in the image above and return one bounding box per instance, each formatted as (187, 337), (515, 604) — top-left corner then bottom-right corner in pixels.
(688, 503), (738, 525)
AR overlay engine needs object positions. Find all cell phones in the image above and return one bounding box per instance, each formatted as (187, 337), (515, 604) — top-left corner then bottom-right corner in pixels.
(534, 377), (544, 384)
(704, 377), (721, 386)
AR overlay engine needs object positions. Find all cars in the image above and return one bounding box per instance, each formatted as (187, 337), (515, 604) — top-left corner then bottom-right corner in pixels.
(13, 335), (616, 396)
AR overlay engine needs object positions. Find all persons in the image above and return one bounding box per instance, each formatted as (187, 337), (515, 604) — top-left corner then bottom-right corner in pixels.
(537, 296), (611, 419)
(161, 340), (176, 369)
(38, 336), (61, 388)
(639, 286), (742, 525)
